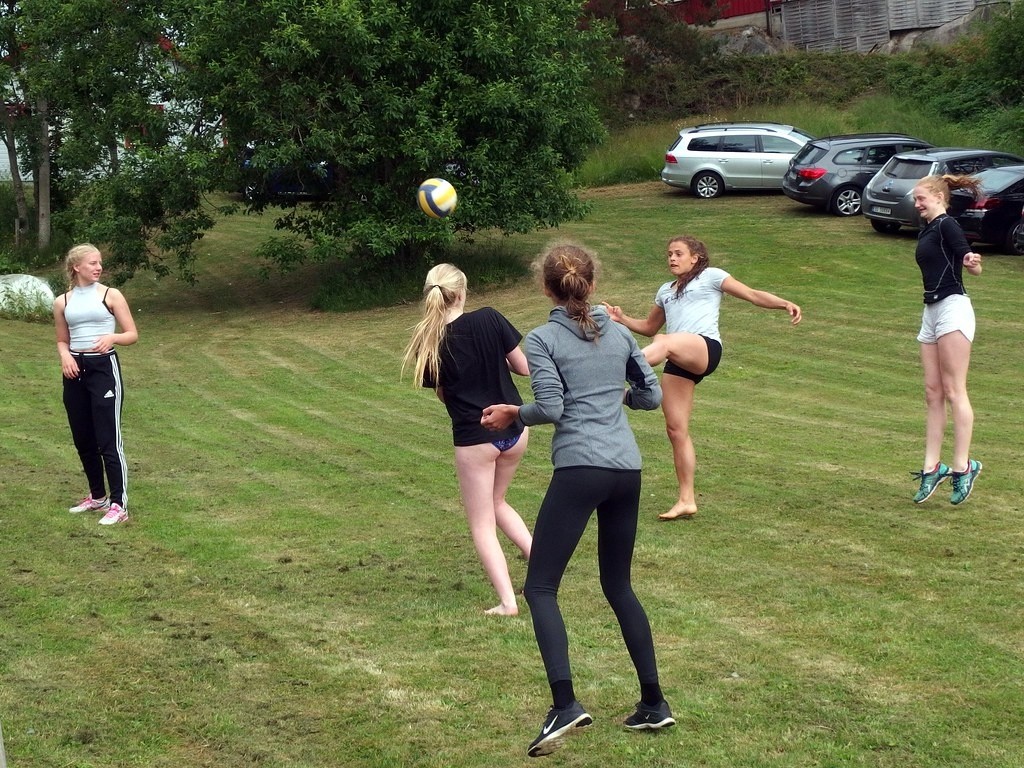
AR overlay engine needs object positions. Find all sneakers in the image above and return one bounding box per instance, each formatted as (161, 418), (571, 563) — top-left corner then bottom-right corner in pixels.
(910, 462), (952, 503)
(942, 459), (982, 505)
(68, 493), (111, 513)
(98, 503), (128, 526)
(527, 701), (593, 757)
(623, 700), (676, 731)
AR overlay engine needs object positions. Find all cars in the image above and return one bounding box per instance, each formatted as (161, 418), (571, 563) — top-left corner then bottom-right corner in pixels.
(863, 146), (1024, 255)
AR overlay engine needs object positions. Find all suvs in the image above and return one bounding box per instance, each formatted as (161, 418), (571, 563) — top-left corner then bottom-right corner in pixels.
(783, 133), (940, 215)
(245, 140), (467, 202)
(663, 121), (818, 199)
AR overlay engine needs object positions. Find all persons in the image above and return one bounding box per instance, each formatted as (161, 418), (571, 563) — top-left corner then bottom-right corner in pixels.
(54, 244), (138, 526)
(599, 234), (804, 522)
(479, 245), (675, 756)
(911, 173), (993, 507)
(399, 263), (533, 616)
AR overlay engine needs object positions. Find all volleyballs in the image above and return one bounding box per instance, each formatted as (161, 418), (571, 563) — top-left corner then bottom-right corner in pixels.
(416, 177), (459, 219)
